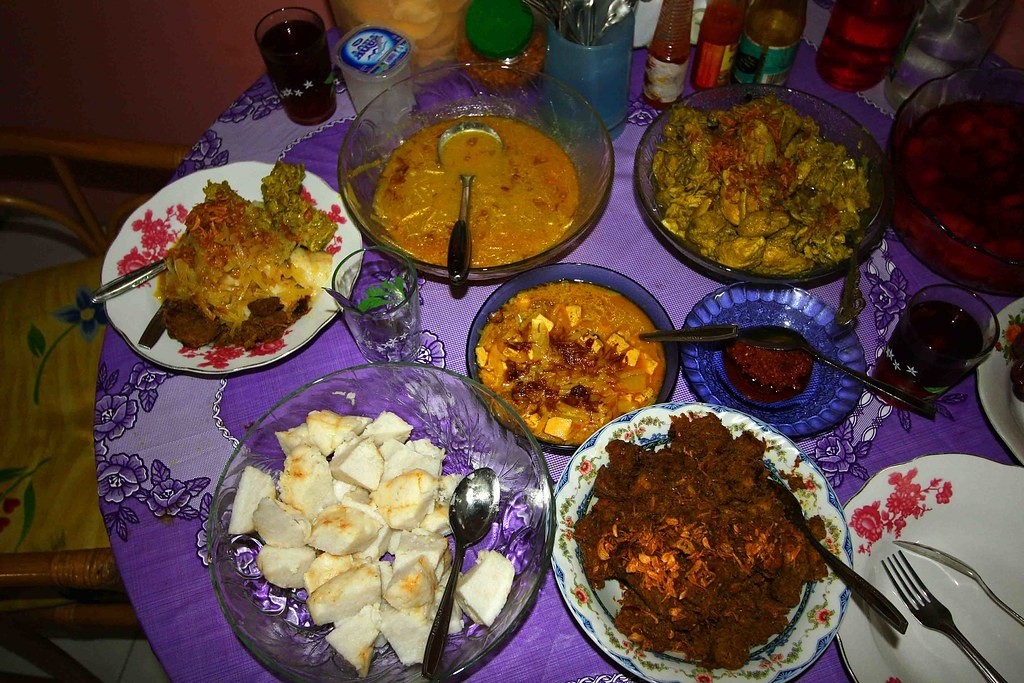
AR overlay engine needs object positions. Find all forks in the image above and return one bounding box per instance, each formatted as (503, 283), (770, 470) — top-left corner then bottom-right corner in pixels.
(522, 0), (639, 47)
(881, 550), (1007, 683)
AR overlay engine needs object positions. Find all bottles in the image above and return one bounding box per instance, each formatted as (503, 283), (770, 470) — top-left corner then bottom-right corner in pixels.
(815, 1), (922, 92)
(883, 0), (1016, 114)
(453, 0), (546, 97)
(690, 0), (749, 91)
(730, 0), (808, 87)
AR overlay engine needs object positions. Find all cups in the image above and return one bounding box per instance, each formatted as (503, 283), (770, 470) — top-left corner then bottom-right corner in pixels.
(543, 8), (635, 131)
(871, 284), (1000, 411)
(254, 6), (337, 126)
(331, 246), (423, 366)
(340, 21), (416, 125)
(642, 0), (694, 110)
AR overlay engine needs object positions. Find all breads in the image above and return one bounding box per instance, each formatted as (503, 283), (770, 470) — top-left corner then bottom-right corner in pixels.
(229, 409), (516, 677)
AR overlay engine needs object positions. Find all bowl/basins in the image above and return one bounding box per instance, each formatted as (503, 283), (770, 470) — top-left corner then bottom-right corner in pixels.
(633, 84), (894, 285)
(884, 67), (1024, 297)
(207, 363), (558, 683)
(337, 63), (614, 281)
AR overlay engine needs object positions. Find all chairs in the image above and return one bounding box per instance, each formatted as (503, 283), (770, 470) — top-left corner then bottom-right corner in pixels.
(0, 125), (192, 683)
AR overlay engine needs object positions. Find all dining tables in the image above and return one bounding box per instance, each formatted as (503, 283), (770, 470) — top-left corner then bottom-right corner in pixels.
(91, 0), (1024, 683)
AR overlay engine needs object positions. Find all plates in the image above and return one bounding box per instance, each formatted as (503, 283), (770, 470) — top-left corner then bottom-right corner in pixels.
(465, 262), (679, 451)
(834, 452), (1023, 683)
(975, 297), (1024, 465)
(547, 401), (853, 683)
(101, 160), (363, 378)
(679, 280), (867, 438)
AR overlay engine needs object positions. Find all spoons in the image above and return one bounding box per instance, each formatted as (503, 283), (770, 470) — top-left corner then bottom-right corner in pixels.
(736, 325), (937, 420)
(437, 121), (503, 300)
(834, 160), (884, 325)
(420, 467), (501, 680)
(765, 475), (908, 636)
(891, 541), (1024, 626)
(322, 286), (403, 339)
